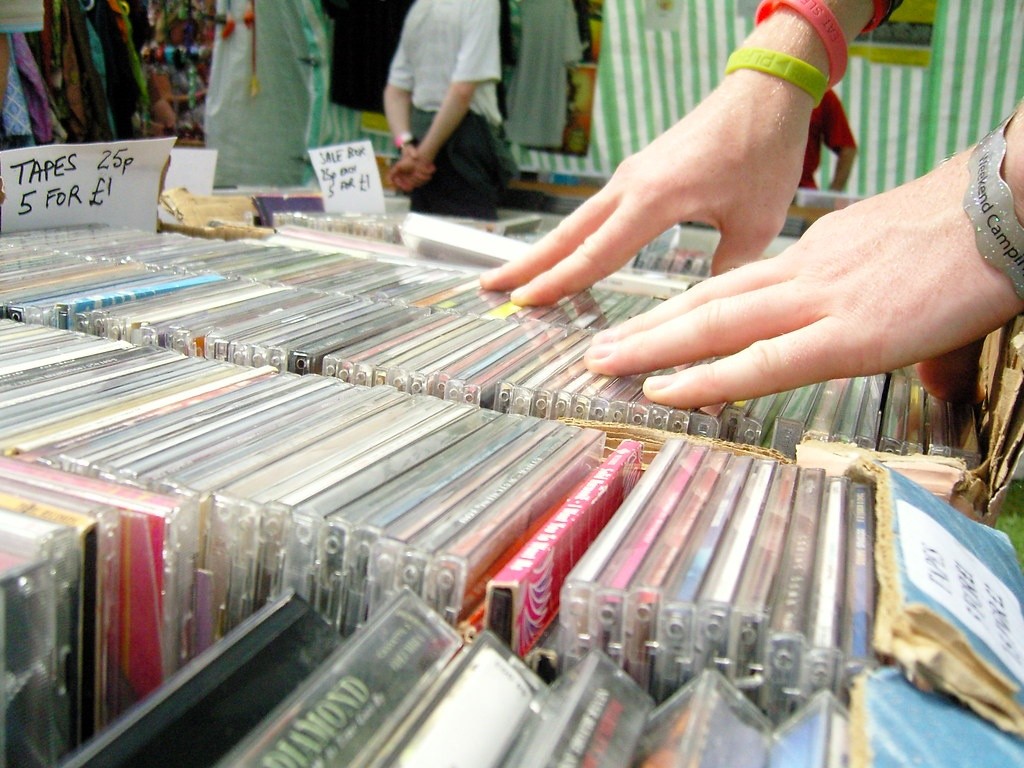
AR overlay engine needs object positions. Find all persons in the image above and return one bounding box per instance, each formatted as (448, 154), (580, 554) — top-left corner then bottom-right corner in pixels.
(201, 0), (308, 190)
(381, 0), (505, 224)
(788, 88), (855, 206)
(480, 0), (1024, 409)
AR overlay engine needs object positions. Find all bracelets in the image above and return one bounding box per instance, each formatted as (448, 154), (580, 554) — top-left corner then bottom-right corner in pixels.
(962, 111), (1024, 302)
(392, 130), (419, 150)
(724, 47), (827, 109)
(860, 0), (904, 35)
(754, 0), (849, 91)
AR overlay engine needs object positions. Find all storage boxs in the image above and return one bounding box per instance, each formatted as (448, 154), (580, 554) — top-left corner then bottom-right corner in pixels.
(159, 188), (1024, 766)
